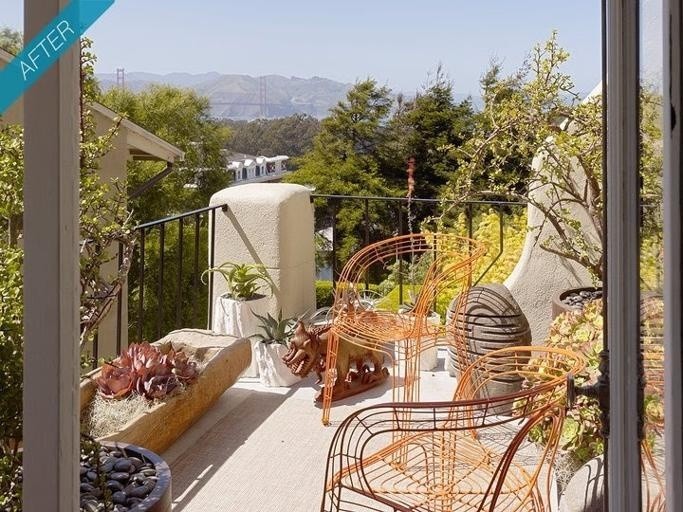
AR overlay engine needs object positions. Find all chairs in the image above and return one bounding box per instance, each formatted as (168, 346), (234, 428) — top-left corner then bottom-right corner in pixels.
(321, 346), (585, 512)
(320, 234), (486, 467)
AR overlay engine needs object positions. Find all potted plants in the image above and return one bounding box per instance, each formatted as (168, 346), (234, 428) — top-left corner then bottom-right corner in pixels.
(463, 30), (662, 426)
(395, 292), (440, 372)
(247, 308), (309, 386)
(0, 35), (173, 512)
(201, 262), (275, 379)
